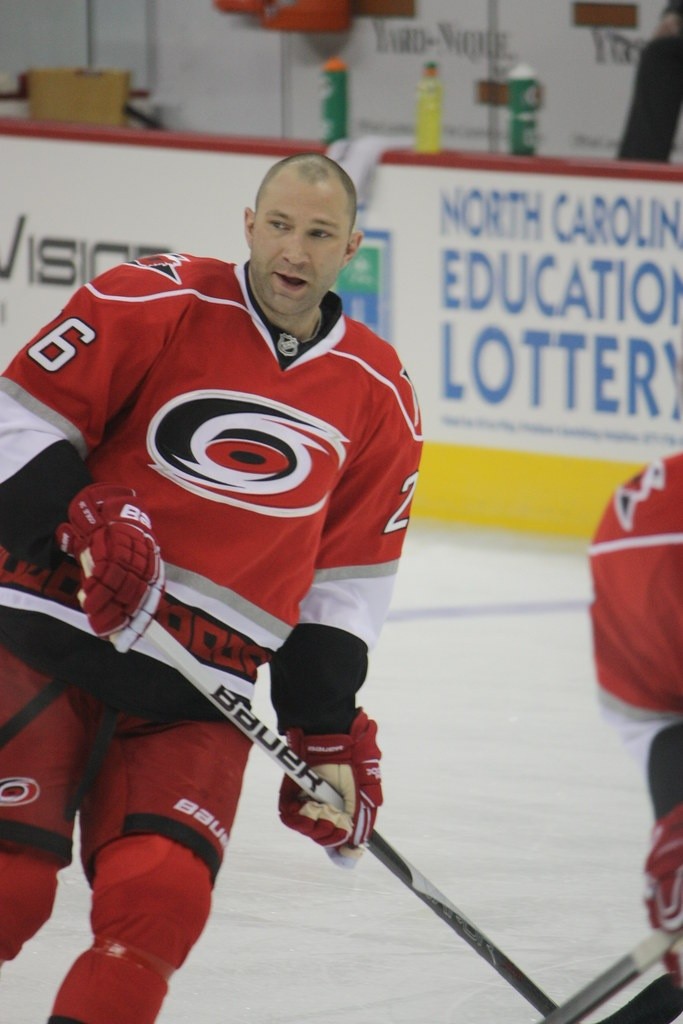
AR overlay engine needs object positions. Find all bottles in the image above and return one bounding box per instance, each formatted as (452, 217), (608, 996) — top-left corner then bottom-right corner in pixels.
(508, 56), (541, 156)
(320, 49), (347, 144)
(417, 60), (442, 153)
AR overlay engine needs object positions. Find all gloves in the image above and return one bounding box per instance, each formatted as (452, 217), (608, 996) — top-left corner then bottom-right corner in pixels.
(278, 707), (383, 868)
(53, 482), (166, 655)
(645, 803), (683, 986)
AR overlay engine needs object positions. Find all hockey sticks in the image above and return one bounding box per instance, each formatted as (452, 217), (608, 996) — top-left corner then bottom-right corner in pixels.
(145, 617), (581, 1024)
(542, 926), (683, 1024)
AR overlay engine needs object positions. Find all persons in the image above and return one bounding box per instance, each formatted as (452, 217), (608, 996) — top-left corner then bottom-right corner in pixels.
(615, 0), (683, 164)
(588, 450), (683, 989)
(0, 153), (424, 1024)
